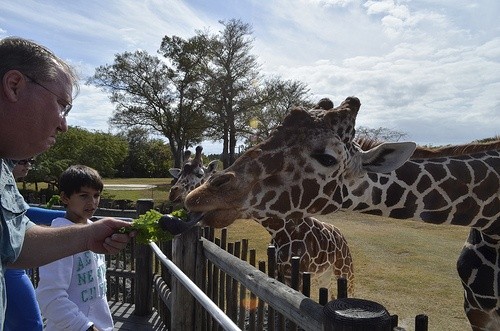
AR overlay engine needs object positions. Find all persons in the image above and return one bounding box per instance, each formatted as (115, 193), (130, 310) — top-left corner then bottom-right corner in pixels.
(0, 38), (136, 331)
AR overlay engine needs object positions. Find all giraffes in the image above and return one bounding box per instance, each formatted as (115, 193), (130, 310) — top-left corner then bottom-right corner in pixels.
(168, 146), (357, 301)
(184, 94), (500, 331)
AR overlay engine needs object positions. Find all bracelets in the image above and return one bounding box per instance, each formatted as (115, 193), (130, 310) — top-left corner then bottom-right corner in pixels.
(86, 324), (94, 331)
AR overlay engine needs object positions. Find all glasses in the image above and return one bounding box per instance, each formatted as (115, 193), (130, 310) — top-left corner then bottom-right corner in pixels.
(9, 69), (73, 119)
(12, 160), (33, 165)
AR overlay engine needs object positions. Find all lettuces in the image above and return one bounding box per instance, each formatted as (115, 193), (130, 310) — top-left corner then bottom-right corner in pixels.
(119, 209), (189, 242)
